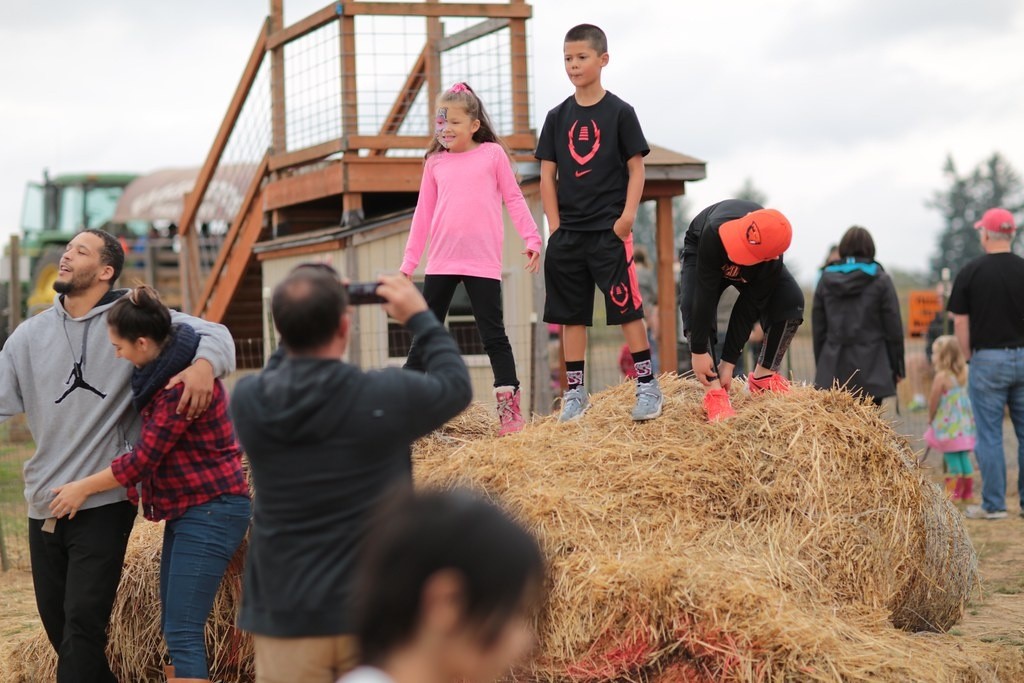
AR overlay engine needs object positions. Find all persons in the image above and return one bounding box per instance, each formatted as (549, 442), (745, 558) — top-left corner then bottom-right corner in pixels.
(947, 208), (1024, 520)
(617, 247), (660, 382)
(926, 281), (955, 368)
(810, 226), (908, 407)
(681, 198), (804, 426)
(50, 284), (252, 682)
(532, 23), (664, 422)
(398, 82), (543, 438)
(228, 254), (473, 683)
(133, 221), (215, 268)
(922, 334), (977, 507)
(0, 229), (236, 683)
(332, 487), (549, 683)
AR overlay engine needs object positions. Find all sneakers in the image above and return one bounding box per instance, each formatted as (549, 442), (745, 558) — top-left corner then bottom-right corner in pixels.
(748, 371), (804, 402)
(632, 377), (663, 420)
(496, 389), (526, 436)
(703, 386), (738, 428)
(966, 505), (1007, 519)
(558, 385), (591, 424)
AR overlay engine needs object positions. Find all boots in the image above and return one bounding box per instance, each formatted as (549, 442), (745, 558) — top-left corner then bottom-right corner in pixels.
(943, 477), (962, 504)
(961, 477), (973, 504)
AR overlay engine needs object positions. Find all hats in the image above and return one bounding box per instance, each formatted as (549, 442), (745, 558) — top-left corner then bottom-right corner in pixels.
(974, 208), (1016, 234)
(718, 208), (792, 265)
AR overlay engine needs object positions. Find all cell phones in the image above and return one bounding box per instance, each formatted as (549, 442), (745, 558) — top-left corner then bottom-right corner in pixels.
(348, 282), (387, 305)
